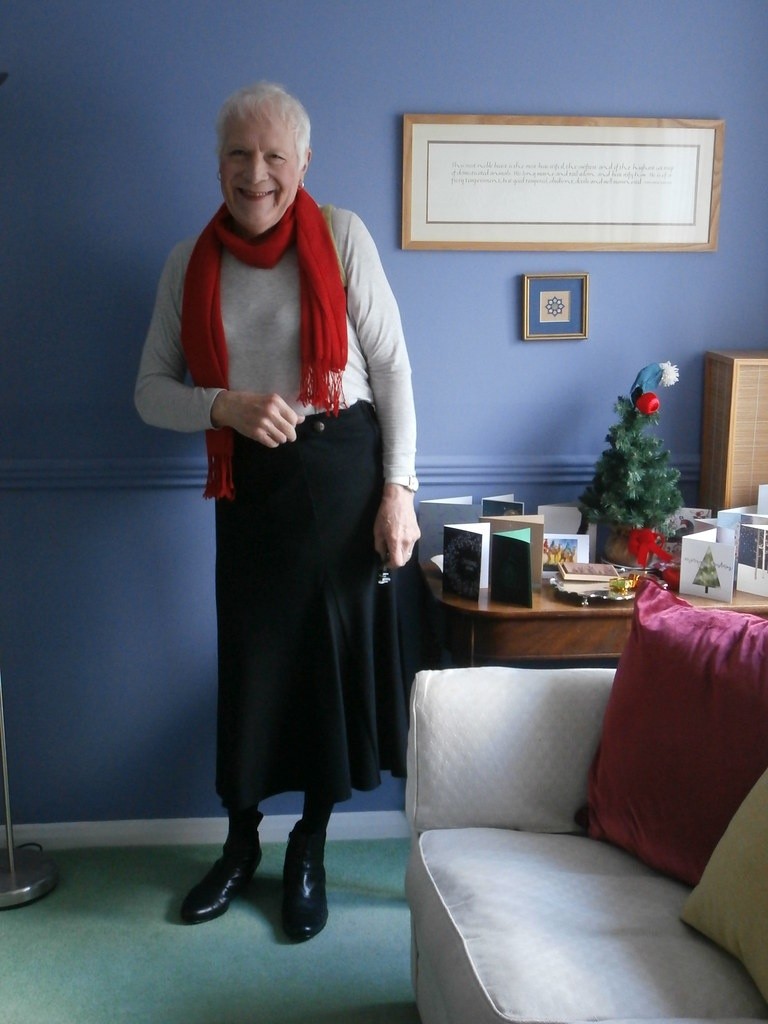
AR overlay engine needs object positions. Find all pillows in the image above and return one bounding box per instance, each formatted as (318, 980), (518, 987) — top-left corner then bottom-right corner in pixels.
(676, 767), (768, 1008)
(573, 576), (768, 887)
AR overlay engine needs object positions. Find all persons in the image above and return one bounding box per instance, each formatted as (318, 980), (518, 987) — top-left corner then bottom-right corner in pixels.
(125, 77), (428, 944)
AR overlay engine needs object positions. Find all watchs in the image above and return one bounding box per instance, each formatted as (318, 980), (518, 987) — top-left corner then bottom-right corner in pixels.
(382, 472), (421, 493)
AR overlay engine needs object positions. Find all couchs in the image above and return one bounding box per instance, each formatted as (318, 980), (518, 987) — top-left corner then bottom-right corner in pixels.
(405, 665), (768, 1024)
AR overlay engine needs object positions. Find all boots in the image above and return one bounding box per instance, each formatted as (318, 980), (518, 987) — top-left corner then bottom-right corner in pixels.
(180, 810), (264, 926)
(279, 820), (331, 946)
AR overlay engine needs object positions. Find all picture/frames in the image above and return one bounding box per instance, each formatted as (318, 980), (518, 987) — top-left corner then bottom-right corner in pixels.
(521, 272), (589, 341)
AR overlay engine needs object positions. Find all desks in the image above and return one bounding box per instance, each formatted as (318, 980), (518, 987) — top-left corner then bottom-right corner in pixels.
(419, 559), (768, 669)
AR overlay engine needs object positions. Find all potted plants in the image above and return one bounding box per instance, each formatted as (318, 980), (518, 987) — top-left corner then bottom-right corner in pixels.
(578, 362), (685, 566)
